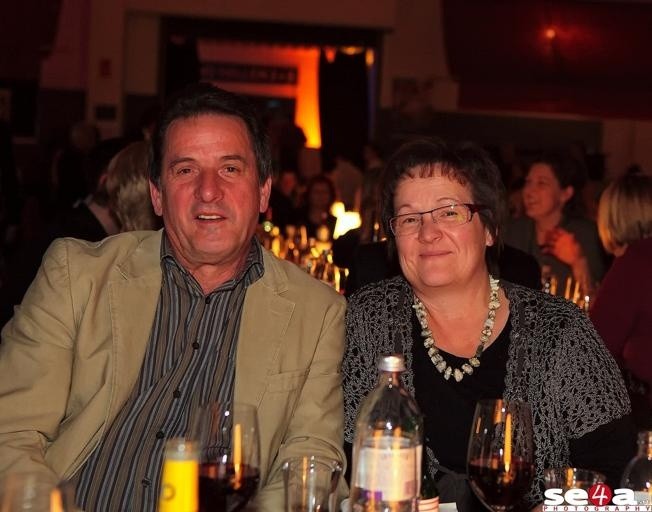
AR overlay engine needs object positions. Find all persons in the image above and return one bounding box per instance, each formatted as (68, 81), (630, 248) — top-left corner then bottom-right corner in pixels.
(0, 80), (651, 510)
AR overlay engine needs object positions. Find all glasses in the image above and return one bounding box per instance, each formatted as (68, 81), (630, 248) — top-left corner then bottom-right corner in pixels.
(388, 203), (486, 237)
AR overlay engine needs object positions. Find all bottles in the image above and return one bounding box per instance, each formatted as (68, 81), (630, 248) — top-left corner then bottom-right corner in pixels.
(157, 440), (198, 512)
(624, 431), (652, 493)
(349, 355), (421, 511)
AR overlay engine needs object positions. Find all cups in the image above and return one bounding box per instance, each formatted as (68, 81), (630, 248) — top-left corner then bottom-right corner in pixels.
(198, 402), (259, 512)
(465, 398), (537, 511)
(284, 457), (334, 511)
(1, 478), (62, 512)
